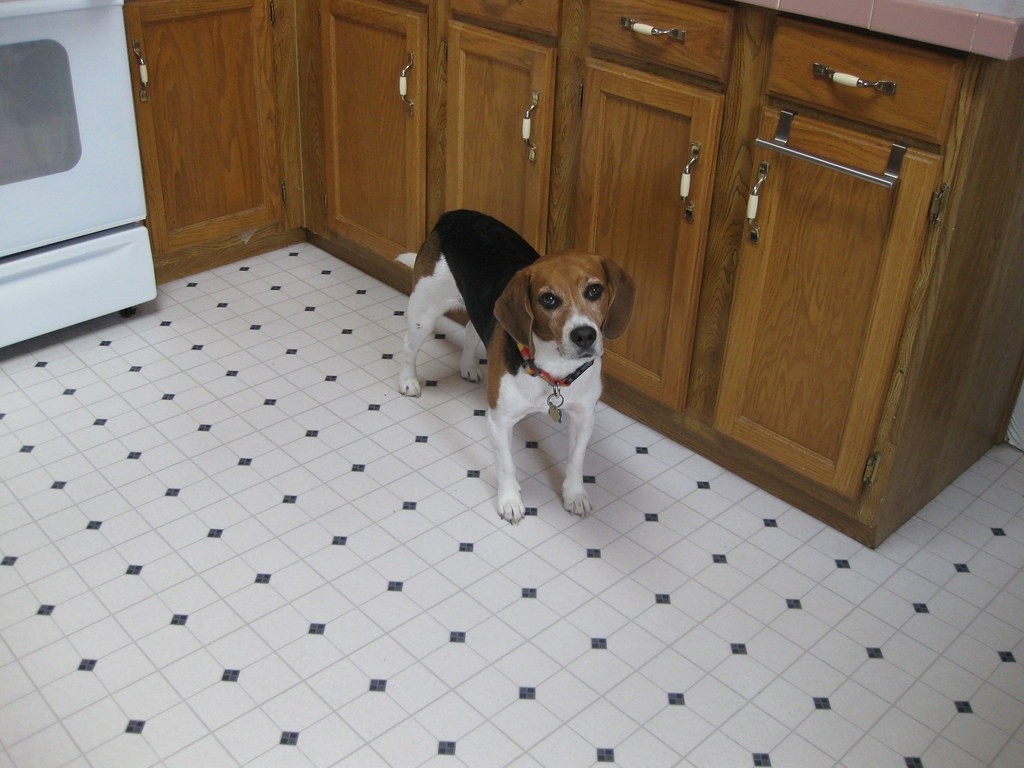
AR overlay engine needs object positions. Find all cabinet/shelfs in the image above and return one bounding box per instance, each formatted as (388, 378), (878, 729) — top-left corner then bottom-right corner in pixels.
(124, 0), (1024, 551)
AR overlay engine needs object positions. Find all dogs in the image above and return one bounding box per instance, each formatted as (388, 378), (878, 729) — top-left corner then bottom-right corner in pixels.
(393, 208), (637, 526)
